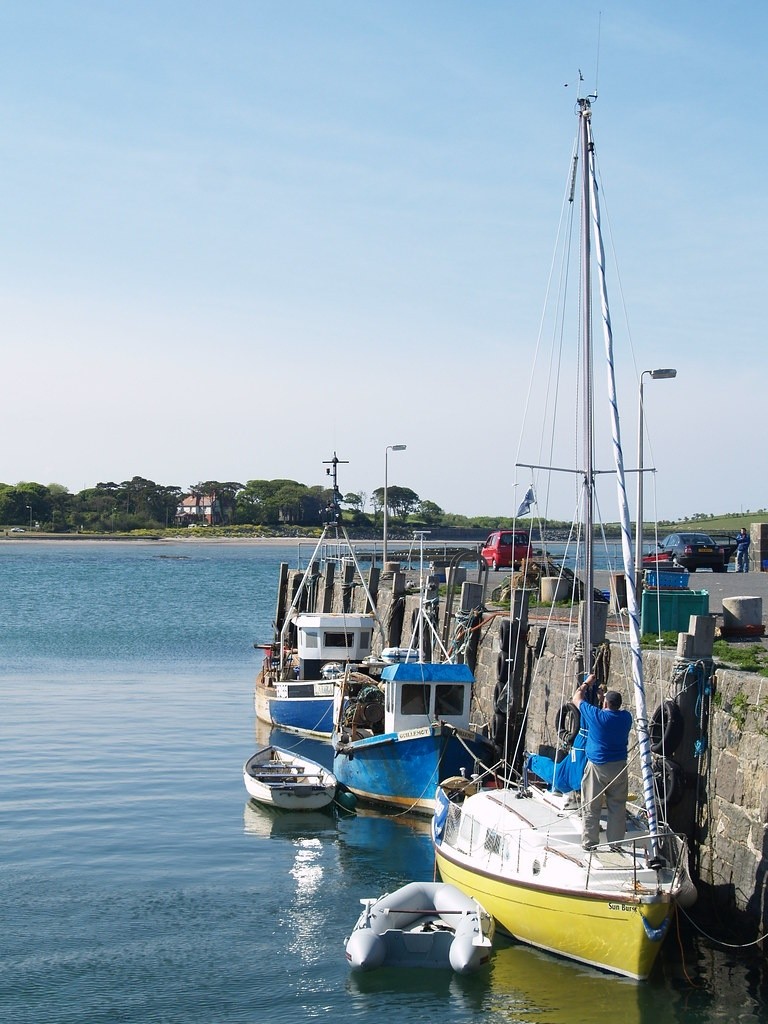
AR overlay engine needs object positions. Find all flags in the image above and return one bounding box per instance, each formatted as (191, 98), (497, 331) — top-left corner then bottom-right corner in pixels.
(516, 486), (536, 517)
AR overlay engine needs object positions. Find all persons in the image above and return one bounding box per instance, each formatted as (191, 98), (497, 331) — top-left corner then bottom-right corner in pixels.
(573, 673), (634, 854)
(735, 527), (751, 573)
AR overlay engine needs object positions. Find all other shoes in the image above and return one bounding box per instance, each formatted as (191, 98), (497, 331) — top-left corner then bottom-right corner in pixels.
(735, 570), (749, 573)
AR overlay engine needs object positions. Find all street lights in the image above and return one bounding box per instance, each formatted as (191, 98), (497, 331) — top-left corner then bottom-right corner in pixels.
(635, 368), (677, 605)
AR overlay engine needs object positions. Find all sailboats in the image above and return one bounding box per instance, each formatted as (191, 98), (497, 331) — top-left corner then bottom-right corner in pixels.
(432, 89), (700, 982)
(382, 444), (407, 577)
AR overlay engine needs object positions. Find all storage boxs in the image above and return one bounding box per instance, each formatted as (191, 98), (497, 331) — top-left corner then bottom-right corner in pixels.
(637, 559), (711, 639)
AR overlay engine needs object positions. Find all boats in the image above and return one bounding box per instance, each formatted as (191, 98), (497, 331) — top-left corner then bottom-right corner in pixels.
(345, 878), (497, 975)
(241, 745), (336, 810)
(331, 529), (501, 817)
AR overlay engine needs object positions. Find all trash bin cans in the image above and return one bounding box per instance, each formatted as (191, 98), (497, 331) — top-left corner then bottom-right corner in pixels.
(639, 588), (710, 639)
(429, 561), (456, 583)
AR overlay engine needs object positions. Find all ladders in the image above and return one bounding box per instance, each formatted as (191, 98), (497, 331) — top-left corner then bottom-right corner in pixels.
(548, 562), (610, 605)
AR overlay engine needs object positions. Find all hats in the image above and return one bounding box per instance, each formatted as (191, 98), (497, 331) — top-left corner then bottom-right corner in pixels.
(603, 691), (623, 704)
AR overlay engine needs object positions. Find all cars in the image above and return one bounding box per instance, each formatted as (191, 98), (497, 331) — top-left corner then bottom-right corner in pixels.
(252, 505), (390, 743)
(653, 533), (739, 573)
(479, 531), (533, 572)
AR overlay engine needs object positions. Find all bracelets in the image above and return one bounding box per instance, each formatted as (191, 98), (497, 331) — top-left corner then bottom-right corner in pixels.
(583, 680), (590, 687)
